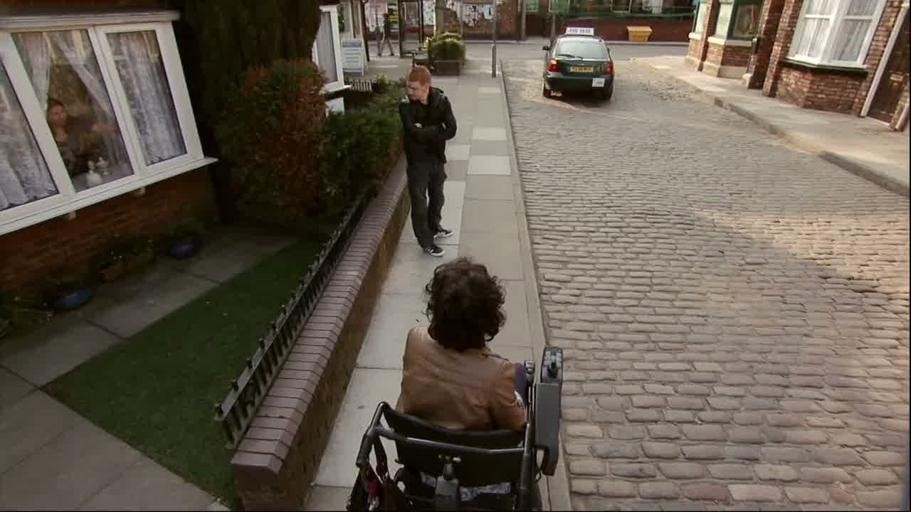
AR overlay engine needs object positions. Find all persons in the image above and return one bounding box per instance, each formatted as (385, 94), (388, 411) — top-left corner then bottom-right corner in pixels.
(395, 63), (457, 258)
(377, 12), (395, 57)
(44, 96), (119, 178)
(397, 253), (529, 503)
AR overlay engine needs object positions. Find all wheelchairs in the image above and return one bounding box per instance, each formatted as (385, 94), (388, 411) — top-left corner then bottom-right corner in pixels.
(344, 343), (569, 509)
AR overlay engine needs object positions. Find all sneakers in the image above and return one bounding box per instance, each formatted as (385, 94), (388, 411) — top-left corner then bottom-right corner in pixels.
(425, 243), (445, 256)
(436, 230), (454, 238)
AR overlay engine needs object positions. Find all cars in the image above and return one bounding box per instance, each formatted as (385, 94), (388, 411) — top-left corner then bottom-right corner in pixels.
(540, 25), (617, 106)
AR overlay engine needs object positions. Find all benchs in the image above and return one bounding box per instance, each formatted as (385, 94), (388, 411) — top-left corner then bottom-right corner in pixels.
(411, 39), (430, 67)
